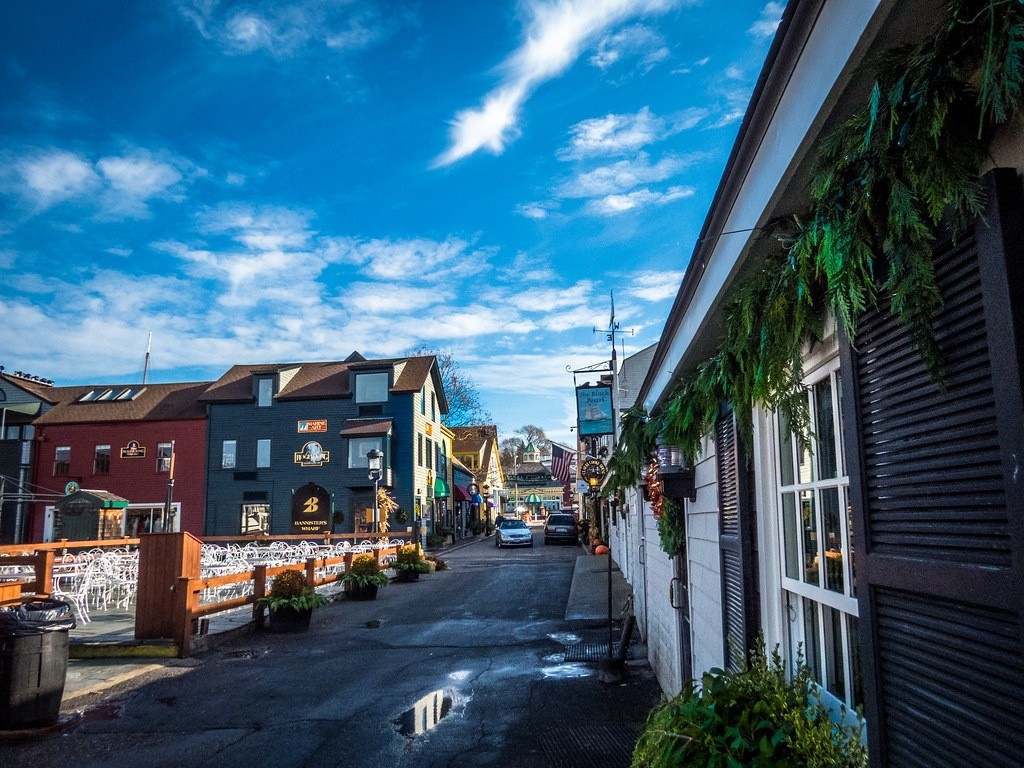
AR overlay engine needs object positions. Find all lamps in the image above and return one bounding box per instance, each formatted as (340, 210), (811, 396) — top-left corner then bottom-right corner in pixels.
(655, 464), (697, 502)
(638, 483), (652, 502)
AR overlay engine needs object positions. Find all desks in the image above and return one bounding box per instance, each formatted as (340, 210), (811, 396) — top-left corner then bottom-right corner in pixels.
(52, 562), (87, 572)
(242, 558), (287, 566)
(200, 565), (227, 578)
(0, 572), (83, 595)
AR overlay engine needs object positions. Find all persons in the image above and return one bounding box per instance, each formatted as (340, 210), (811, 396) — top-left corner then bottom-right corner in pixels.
(495, 512), (504, 528)
(128, 517), (145, 538)
(153, 517), (162, 532)
(143, 516), (150, 532)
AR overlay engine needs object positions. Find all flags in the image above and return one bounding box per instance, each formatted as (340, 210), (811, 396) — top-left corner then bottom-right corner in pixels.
(552, 443), (573, 482)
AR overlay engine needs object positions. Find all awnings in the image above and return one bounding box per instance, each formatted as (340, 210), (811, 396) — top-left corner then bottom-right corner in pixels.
(524, 494), (542, 503)
(455, 484), (472, 501)
(486, 500), (497, 507)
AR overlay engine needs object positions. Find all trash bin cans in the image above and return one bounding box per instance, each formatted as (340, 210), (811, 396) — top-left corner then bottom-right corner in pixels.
(0, 602), (73, 730)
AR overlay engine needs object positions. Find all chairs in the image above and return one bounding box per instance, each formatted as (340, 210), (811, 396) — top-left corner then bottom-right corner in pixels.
(0, 538), (407, 626)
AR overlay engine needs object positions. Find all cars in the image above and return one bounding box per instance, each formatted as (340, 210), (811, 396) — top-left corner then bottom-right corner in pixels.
(496, 520), (533, 548)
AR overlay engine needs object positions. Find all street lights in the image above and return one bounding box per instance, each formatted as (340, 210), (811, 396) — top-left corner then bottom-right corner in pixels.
(367, 449), (384, 543)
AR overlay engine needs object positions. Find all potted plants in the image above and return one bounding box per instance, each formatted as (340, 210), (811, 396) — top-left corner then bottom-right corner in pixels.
(334, 555), (390, 602)
(255, 569), (330, 631)
(387, 545), (427, 582)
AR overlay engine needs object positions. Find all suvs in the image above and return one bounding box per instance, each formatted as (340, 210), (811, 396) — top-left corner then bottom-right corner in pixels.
(544, 514), (578, 545)
